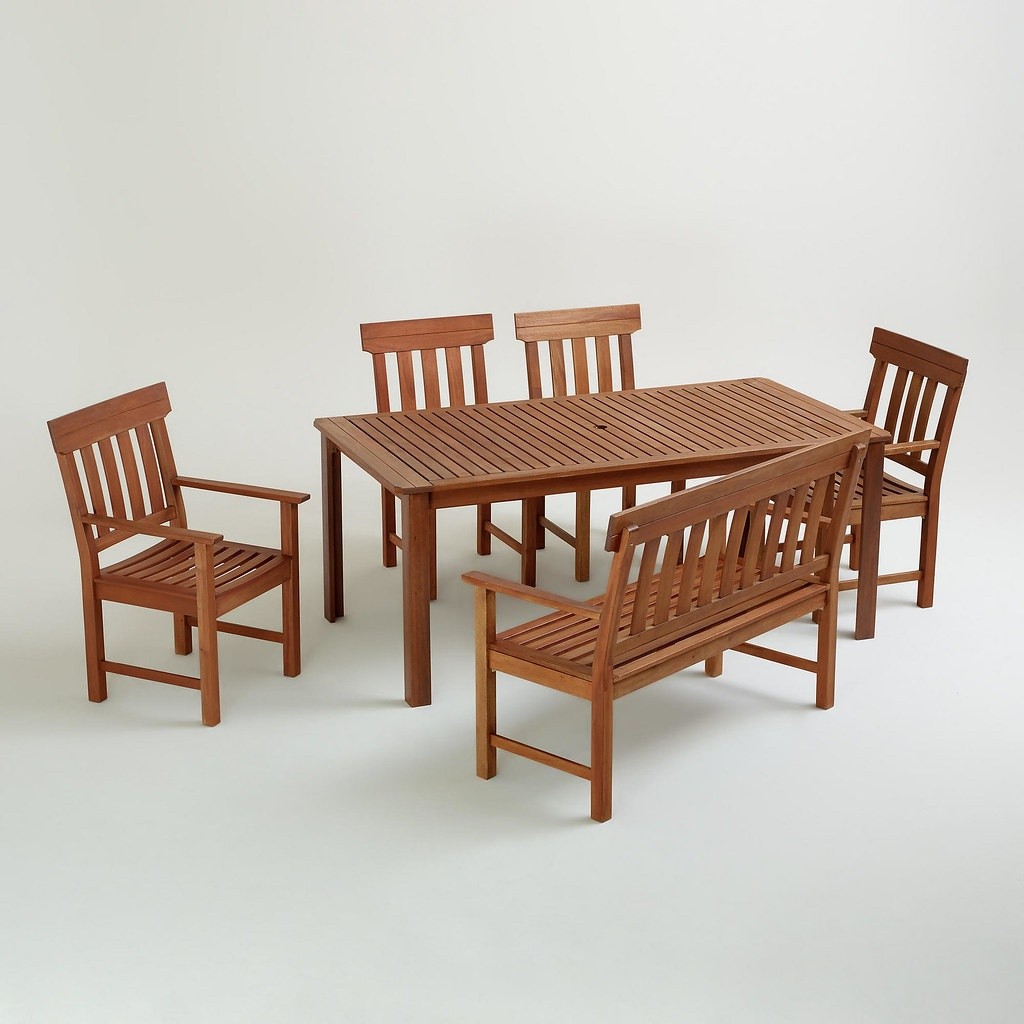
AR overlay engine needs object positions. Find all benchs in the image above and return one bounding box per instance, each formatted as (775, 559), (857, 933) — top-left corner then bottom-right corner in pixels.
(460, 427), (872, 823)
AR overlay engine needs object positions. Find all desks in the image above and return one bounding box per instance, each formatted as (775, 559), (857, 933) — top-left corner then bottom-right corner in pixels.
(313, 377), (892, 708)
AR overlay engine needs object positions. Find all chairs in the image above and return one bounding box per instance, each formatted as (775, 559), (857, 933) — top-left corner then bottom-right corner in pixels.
(360, 314), (537, 599)
(514, 303), (686, 582)
(751, 327), (969, 624)
(46, 380), (311, 726)
